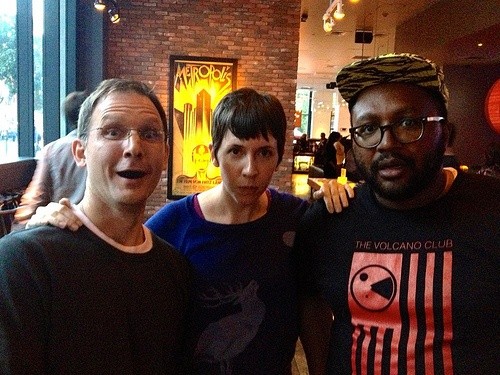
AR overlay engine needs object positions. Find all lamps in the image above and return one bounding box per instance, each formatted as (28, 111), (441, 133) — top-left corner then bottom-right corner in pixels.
(334, 1), (345, 19)
(323, 11), (335, 32)
(94, 0), (120, 23)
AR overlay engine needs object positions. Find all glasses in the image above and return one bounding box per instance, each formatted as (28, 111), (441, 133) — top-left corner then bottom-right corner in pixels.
(349, 113), (445, 149)
(78, 125), (170, 144)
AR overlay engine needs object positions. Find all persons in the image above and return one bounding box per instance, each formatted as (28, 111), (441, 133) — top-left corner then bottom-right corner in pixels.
(300, 132), (345, 178)
(31, 87), (354, 375)
(292, 52), (499, 375)
(1, 79), (190, 375)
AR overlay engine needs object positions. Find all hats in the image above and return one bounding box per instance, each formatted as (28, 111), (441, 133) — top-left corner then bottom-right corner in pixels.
(335, 53), (450, 109)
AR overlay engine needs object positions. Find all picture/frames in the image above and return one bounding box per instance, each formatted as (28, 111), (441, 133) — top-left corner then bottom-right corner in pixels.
(167, 55), (238, 201)
